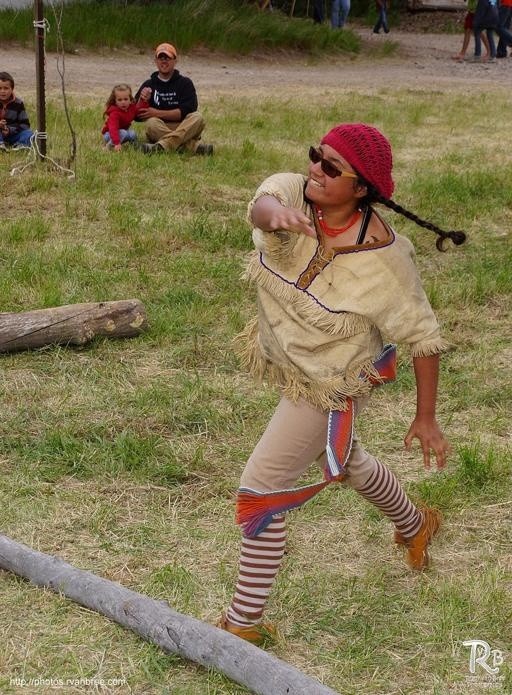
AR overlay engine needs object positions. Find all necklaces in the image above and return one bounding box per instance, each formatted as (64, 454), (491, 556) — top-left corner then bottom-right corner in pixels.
(314, 204), (362, 236)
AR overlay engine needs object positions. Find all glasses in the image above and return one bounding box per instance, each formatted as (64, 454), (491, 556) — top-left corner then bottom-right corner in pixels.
(309, 146), (356, 178)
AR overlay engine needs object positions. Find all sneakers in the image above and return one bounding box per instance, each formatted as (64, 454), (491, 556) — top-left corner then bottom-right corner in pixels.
(394, 508), (439, 568)
(142, 143), (165, 154)
(452, 47), (511, 61)
(197, 142), (215, 156)
(213, 611), (276, 647)
(0, 144), (9, 154)
(10, 142), (32, 155)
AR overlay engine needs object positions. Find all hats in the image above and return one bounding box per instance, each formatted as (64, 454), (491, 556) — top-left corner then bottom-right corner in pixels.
(155, 44), (176, 59)
(323, 124), (394, 199)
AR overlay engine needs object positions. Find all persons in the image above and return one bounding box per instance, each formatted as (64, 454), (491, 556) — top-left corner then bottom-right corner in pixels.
(465, 0), (499, 63)
(372, 0), (390, 34)
(101, 82), (149, 153)
(134, 42), (213, 157)
(330, 0), (351, 27)
(210, 124), (466, 640)
(1, 71), (36, 149)
(493, 0), (511, 57)
(452, 0), (489, 59)
